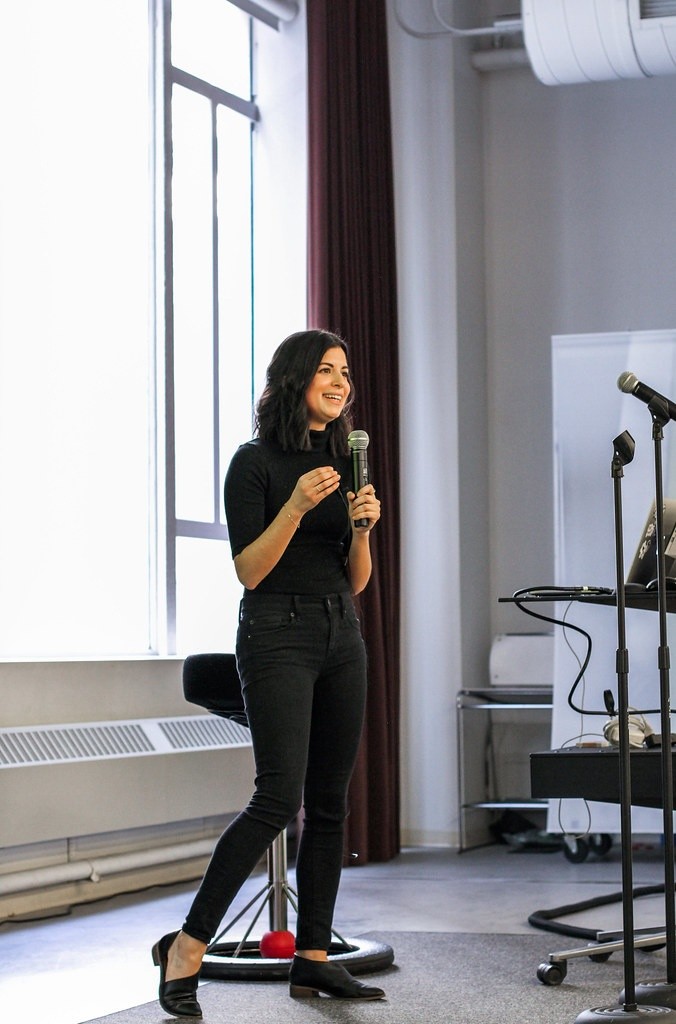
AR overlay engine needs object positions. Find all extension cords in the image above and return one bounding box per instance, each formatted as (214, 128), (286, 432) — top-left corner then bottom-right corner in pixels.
(645, 733), (676, 749)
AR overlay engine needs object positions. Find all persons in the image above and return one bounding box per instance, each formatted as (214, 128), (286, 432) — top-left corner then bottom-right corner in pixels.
(151, 330), (387, 1019)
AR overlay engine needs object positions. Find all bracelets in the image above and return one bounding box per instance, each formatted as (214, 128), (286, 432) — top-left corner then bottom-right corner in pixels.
(283, 504), (300, 529)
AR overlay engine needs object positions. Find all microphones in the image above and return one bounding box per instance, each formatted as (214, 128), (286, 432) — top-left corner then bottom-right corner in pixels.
(617, 371), (676, 421)
(347, 430), (370, 527)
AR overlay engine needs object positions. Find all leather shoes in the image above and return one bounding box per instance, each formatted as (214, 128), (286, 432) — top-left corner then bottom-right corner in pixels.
(151, 928), (202, 1018)
(289, 952), (386, 1002)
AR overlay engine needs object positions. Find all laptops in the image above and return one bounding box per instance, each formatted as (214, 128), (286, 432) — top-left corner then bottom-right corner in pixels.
(526, 496), (676, 596)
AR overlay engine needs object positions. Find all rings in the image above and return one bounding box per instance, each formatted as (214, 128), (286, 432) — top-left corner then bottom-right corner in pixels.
(368, 489), (375, 495)
(316, 486), (321, 491)
